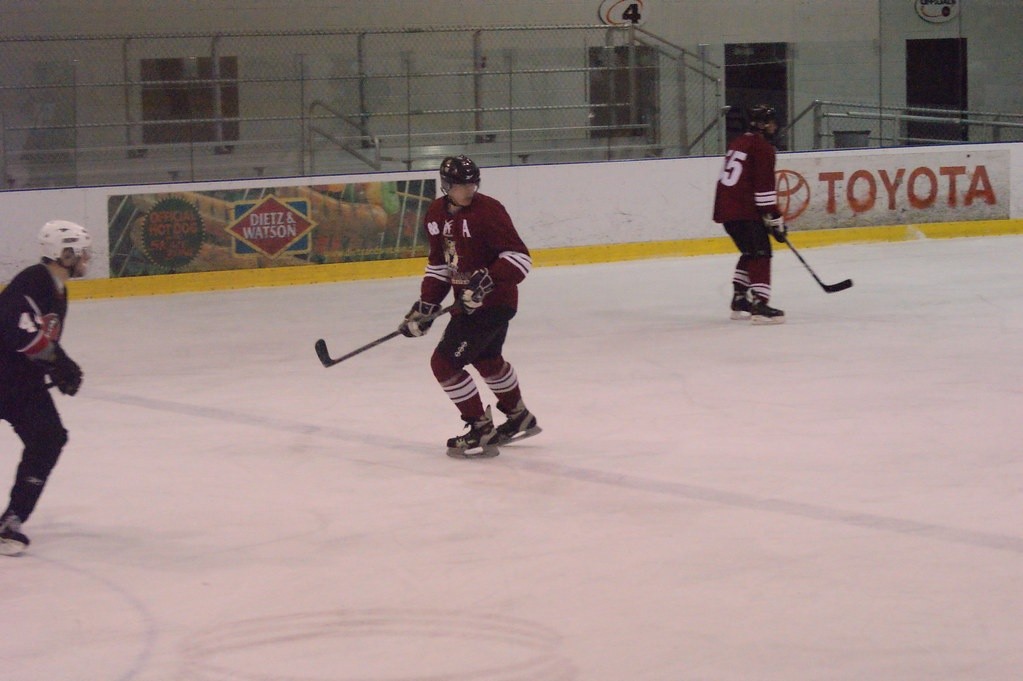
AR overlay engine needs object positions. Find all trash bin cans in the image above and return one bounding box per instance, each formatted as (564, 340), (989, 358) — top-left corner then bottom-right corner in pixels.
(832, 130), (871, 148)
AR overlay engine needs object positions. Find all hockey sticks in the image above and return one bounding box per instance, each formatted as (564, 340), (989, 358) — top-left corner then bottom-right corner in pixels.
(314, 295), (480, 368)
(44, 366), (82, 390)
(783, 236), (854, 294)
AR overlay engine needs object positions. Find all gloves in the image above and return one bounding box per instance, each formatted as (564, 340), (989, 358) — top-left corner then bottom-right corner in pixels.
(398, 301), (442, 337)
(456, 267), (496, 315)
(49, 361), (81, 396)
(763, 212), (787, 243)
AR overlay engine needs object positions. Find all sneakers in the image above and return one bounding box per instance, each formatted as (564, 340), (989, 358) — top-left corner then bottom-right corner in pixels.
(0, 508), (29, 557)
(751, 289), (785, 325)
(446, 404), (500, 460)
(496, 397), (542, 446)
(730, 282), (753, 320)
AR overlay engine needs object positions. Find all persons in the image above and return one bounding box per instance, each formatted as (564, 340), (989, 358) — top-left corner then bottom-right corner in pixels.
(711, 104), (788, 324)
(0, 219), (93, 557)
(397, 155), (543, 462)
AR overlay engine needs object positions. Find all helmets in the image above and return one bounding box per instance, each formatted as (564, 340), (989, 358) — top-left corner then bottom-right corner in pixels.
(439, 155), (480, 185)
(38, 220), (90, 261)
(748, 101), (786, 127)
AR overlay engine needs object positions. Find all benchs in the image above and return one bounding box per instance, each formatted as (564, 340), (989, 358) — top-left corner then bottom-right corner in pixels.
(5, 124), (686, 181)
